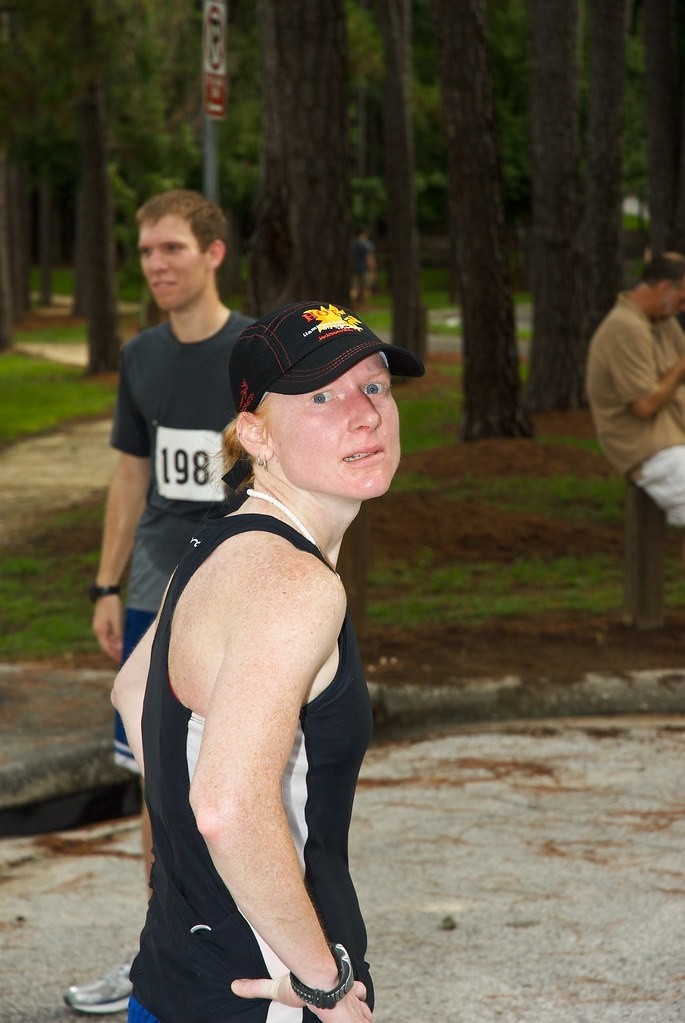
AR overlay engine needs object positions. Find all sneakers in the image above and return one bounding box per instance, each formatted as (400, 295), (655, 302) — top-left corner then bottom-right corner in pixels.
(63, 964), (133, 1015)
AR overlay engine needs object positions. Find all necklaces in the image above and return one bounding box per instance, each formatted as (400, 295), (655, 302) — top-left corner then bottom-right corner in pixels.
(246, 489), (340, 574)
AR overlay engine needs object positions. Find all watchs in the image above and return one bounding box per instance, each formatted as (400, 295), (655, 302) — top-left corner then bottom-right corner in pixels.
(290, 942), (354, 1010)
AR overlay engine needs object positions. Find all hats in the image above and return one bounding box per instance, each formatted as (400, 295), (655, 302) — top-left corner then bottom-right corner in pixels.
(221, 301), (425, 490)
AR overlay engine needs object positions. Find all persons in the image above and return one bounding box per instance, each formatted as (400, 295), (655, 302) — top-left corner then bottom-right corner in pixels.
(109, 302), (403, 1023)
(62, 192), (268, 1018)
(584, 251), (685, 526)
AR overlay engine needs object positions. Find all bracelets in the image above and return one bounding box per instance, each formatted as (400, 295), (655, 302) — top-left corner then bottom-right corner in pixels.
(90, 583), (120, 603)
